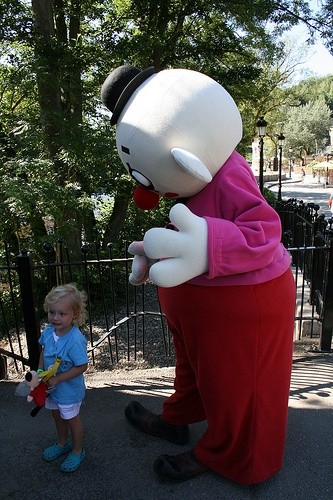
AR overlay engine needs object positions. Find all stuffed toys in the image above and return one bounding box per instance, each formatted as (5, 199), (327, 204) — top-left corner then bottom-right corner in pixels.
(25, 371), (46, 406)
(100, 65), (295, 483)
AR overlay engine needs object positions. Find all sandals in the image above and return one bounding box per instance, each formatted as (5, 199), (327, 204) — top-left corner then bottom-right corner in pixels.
(60, 447), (88, 472)
(43, 438), (73, 461)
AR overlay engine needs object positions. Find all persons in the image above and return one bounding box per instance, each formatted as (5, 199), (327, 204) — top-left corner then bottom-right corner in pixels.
(301, 168), (305, 183)
(38, 285), (88, 471)
(328, 191), (333, 229)
(263, 158), (271, 171)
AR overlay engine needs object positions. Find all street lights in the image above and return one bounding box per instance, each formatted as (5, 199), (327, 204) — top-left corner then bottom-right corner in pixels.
(289, 146), (293, 178)
(256, 116), (269, 195)
(276, 132), (285, 201)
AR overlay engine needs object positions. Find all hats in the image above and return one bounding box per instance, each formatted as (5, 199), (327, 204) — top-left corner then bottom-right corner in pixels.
(101, 64), (154, 127)
